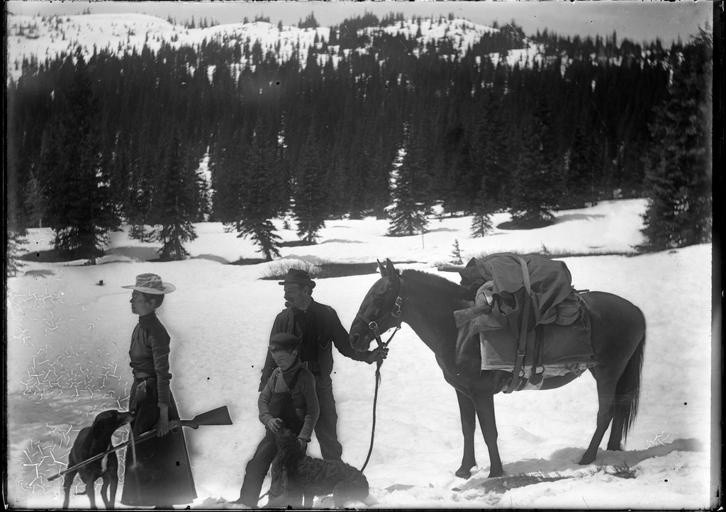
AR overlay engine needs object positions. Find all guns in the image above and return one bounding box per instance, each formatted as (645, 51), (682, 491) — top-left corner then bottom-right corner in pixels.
(46, 406), (233, 482)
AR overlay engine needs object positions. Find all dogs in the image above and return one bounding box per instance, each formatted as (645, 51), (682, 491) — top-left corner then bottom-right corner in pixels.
(62, 410), (136, 510)
(259, 420), (369, 509)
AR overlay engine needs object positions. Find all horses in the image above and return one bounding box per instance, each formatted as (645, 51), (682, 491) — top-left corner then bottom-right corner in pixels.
(347, 255), (646, 479)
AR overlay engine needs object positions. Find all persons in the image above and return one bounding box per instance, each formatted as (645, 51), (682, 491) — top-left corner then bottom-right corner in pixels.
(118, 269), (198, 510)
(261, 266), (390, 460)
(231, 333), (318, 506)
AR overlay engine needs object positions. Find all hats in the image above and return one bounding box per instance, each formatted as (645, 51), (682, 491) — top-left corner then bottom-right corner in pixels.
(270, 332), (299, 348)
(279, 269), (315, 288)
(122, 273), (175, 295)
